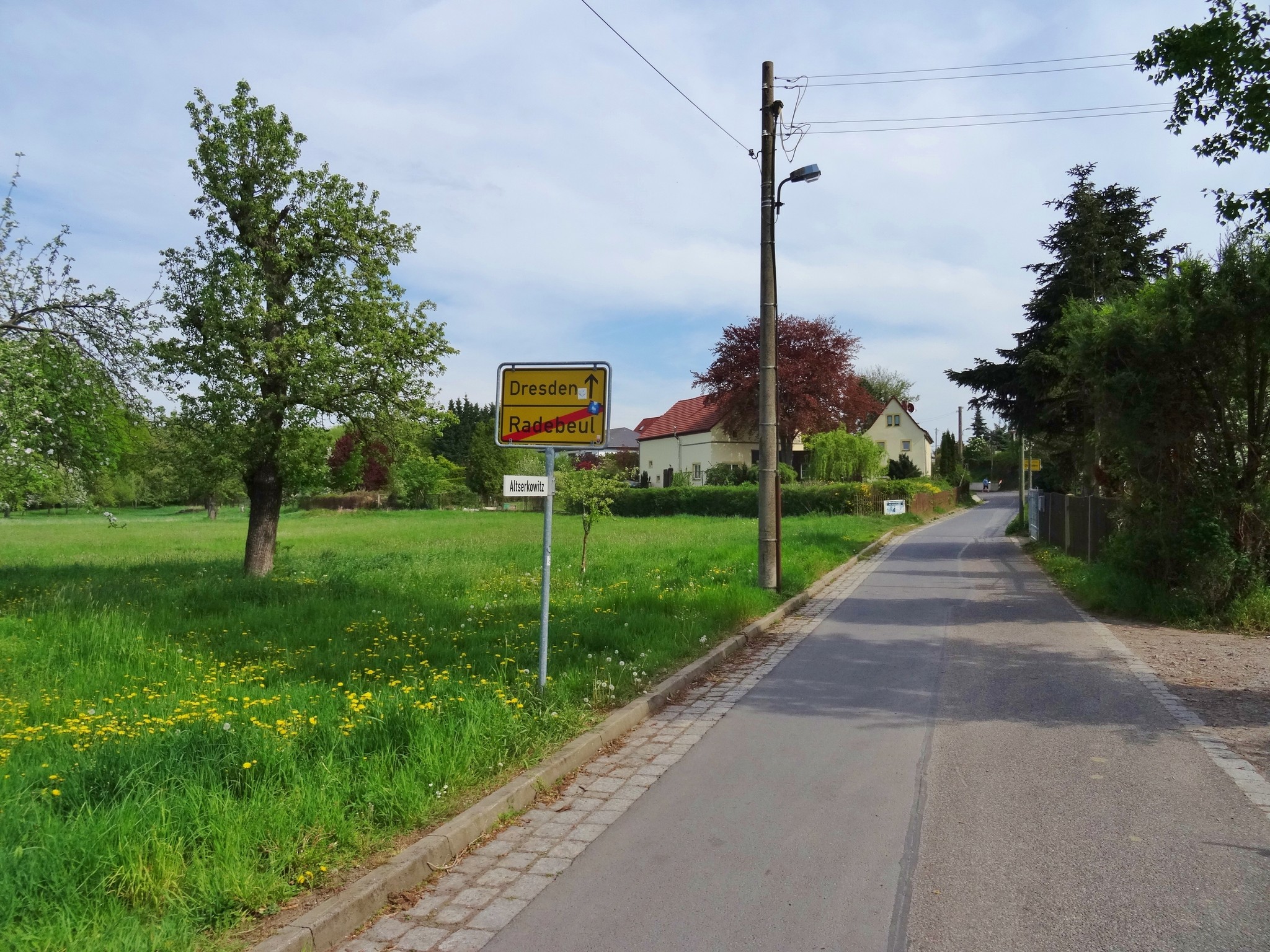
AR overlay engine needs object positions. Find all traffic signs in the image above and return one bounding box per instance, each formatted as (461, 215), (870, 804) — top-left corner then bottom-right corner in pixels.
(495, 361), (612, 452)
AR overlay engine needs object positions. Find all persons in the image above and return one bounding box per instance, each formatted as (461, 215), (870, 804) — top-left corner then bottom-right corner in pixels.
(982, 477), (990, 493)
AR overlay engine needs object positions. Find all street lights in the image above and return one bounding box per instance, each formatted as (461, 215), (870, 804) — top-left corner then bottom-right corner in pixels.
(758, 164), (821, 587)
(959, 428), (971, 465)
